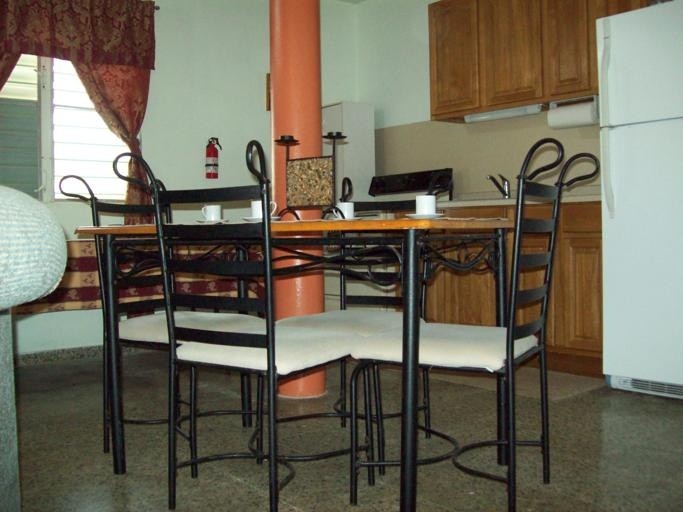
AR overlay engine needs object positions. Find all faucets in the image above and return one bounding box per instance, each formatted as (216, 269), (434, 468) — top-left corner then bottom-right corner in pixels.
(486, 174), (510, 199)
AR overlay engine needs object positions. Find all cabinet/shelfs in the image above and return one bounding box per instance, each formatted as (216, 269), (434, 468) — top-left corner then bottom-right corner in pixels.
(427, 0), (547, 121)
(394, 200), (507, 328)
(550, 0), (673, 104)
(514, 195), (603, 381)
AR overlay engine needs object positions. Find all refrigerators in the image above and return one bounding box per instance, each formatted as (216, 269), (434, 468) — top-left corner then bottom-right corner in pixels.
(592, 3), (682, 401)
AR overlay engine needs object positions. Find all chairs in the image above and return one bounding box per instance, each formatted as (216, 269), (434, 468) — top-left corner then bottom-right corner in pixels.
(334, 168), (453, 474)
(59, 175), (269, 478)
(113, 140), (388, 512)
(349, 137), (599, 512)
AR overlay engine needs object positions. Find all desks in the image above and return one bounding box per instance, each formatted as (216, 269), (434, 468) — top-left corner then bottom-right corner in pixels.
(75, 219), (516, 512)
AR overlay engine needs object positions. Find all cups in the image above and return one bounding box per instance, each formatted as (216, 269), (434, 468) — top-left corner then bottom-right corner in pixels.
(199, 204), (223, 224)
(250, 199), (277, 218)
(414, 194), (438, 214)
(331, 202), (355, 218)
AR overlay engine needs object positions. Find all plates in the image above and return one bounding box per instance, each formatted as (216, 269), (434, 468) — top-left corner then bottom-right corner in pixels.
(403, 213), (444, 220)
(240, 216), (280, 223)
(330, 217), (360, 220)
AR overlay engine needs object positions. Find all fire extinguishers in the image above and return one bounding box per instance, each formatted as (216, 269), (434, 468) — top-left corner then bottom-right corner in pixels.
(205, 136), (222, 179)
